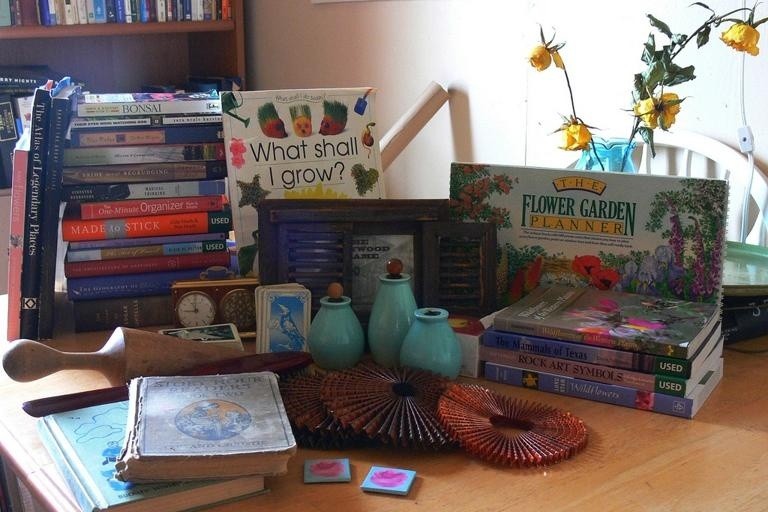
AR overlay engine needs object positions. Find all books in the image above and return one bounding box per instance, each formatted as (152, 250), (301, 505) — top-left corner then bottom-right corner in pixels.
(1, 1), (235, 344)
(38, 402), (271, 511)
(116, 369), (293, 482)
(484, 282), (726, 421)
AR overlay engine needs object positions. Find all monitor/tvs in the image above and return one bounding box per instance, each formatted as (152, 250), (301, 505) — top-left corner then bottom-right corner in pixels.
(38, 402), (271, 511)
(484, 282), (726, 421)
(116, 369), (293, 482)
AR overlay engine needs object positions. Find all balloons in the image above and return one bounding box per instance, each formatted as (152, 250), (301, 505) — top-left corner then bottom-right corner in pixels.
(170, 278), (261, 339)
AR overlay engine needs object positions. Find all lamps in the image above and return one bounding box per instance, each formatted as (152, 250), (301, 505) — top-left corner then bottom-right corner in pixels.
(559, 126), (768, 247)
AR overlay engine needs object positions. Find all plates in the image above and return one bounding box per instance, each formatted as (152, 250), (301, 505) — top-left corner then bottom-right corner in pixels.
(0, 0), (246, 295)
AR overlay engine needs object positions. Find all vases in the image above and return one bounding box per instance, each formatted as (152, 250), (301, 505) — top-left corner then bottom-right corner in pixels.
(530, 1), (768, 172)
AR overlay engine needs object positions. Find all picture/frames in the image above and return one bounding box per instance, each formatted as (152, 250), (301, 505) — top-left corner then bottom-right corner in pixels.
(1, 333), (768, 512)
